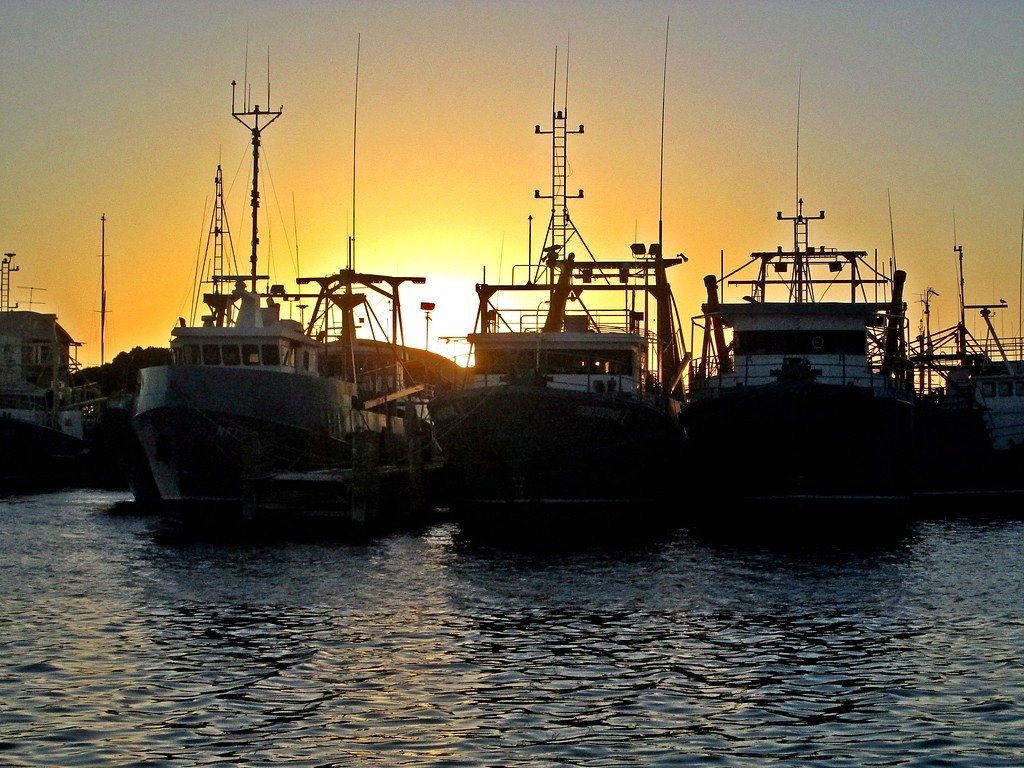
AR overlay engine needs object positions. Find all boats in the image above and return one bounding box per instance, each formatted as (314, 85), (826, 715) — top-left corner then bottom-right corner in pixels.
(133, 13), (1024, 557)
(0, 251), (109, 482)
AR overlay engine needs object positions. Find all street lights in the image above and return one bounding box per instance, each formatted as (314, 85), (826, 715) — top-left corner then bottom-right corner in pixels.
(419, 302), (436, 351)
(630, 241), (664, 372)
(99, 214), (108, 368)
(348, 236), (355, 271)
